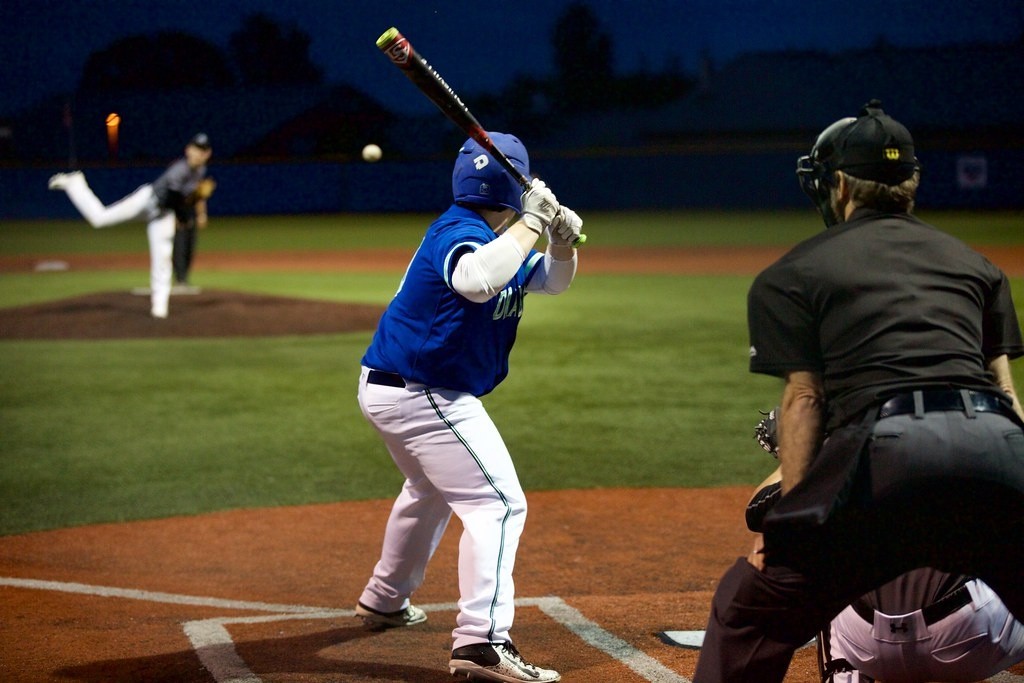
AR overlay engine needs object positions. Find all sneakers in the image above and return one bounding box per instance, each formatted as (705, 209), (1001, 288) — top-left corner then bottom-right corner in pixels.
(356, 598), (427, 624)
(449, 638), (561, 682)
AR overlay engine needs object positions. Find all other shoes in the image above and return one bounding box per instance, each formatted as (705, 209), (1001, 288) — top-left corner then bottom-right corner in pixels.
(50, 170), (84, 189)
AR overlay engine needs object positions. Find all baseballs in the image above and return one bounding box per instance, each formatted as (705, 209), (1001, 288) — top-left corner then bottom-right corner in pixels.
(363, 144), (382, 163)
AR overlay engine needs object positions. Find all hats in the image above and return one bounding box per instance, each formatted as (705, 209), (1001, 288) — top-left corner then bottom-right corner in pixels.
(818, 111), (920, 187)
(189, 131), (211, 150)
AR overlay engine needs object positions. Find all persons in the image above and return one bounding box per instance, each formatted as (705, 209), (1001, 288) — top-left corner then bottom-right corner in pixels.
(689, 116), (1024, 683)
(355, 131), (582, 683)
(49, 133), (211, 318)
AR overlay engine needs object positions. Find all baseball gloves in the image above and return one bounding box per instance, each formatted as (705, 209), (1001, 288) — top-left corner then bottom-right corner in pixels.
(755, 408), (779, 461)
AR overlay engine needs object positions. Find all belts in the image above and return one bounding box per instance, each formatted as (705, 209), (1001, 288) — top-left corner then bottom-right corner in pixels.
(366, 368), (407, 387)
(851, 584), (973, 626)
(830, 388), (1024, 434)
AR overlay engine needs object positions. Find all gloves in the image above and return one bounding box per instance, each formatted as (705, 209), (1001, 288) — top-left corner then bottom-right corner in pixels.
(549, 205), (583, 247)
(518, 176), (556, 233)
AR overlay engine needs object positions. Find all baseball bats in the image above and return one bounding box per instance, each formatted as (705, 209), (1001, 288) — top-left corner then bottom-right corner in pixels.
(376, 25), (588, 250)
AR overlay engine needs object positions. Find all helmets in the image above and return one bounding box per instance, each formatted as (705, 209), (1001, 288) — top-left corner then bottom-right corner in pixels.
(451, 130), (528, 212)
